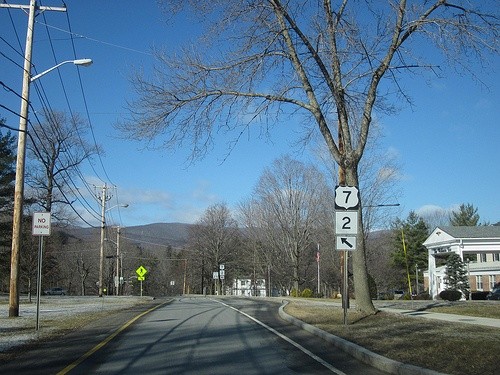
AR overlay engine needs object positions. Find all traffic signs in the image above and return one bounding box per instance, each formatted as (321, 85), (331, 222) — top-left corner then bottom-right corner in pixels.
(31, 212), (50, 236)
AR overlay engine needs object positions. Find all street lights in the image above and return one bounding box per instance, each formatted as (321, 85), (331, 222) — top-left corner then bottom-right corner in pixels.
(8, 57), (93, 316)
(98, 203), (129, 297)
(103, 236), (119, 295)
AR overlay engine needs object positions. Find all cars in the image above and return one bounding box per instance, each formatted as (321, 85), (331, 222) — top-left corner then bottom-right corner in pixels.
(44, 287), (65, 295)
(379, 289), (424, 299)
(486, 288), (500, 300)
(457, 290), (466, 300)
